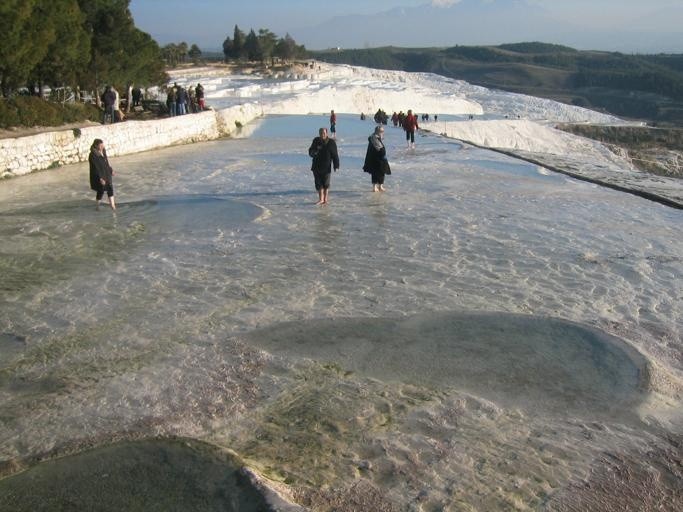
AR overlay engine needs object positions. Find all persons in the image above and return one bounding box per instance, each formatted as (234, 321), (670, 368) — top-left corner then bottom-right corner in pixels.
(89, 138), (116, 211)
(309, 110), (339, 206)
(101, 85), (116, 124)
(167, 81), (205, 117)
(131, 87), (140, 106)
(360, 109), (438, 193)
